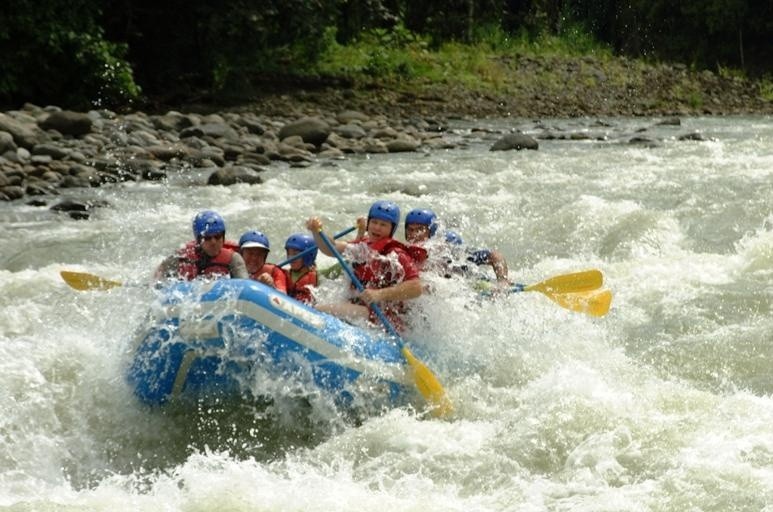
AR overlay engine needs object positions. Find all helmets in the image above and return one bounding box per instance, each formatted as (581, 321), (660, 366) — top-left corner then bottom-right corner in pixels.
(405, 207), (437, 240)
(284, 233), (317, 266)
(442, 230), (462, 246)
(193, 210), (225, 241)
(367, 200), (400, 238)
(468, 249), (493, 265)
(239, 230), (270, 253)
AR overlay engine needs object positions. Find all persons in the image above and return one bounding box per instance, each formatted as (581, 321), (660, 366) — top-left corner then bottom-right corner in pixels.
(305, 201), (423, 331)
(437, 228), (511, 301)
(279, 231), (318, 307)
(240, 232), (288, 297)
(356, 203), (452, 302)
(153, 209), (250, 290)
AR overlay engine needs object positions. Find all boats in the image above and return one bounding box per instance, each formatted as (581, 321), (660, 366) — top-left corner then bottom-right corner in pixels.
(122, 271), (544, 433)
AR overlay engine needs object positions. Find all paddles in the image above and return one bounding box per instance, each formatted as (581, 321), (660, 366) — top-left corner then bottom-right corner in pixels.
(481, 271), (603, 294)
(510, 284), (612, 318)
(62, 270), (166, 293)
(316, 223), (454, 419)
